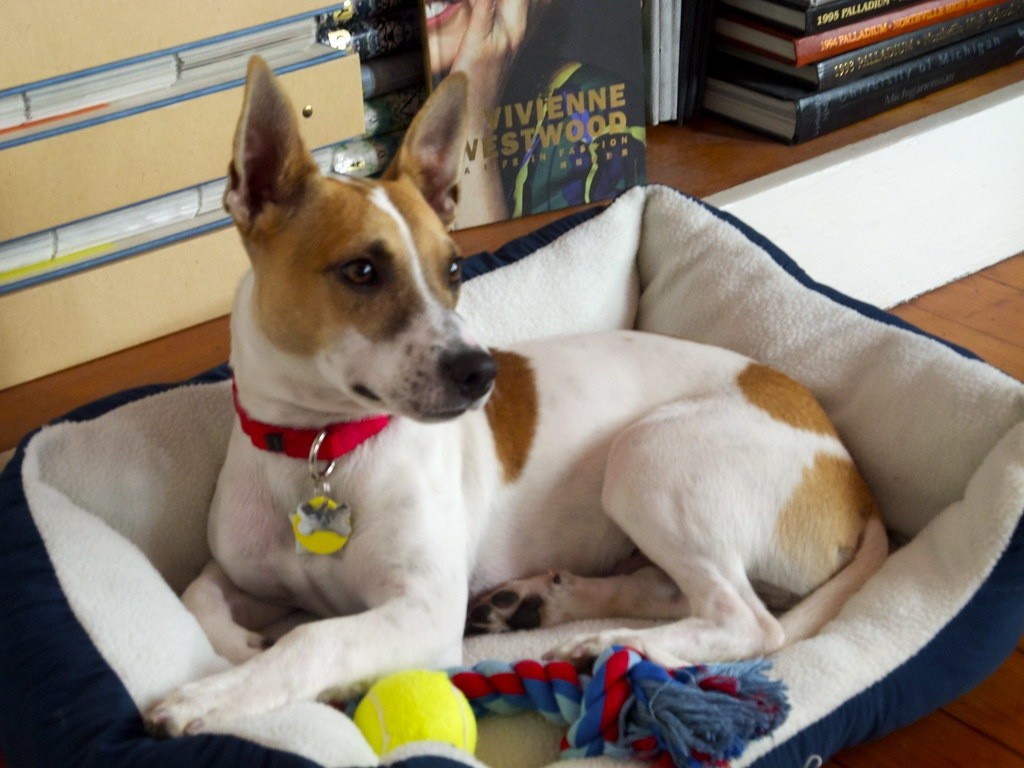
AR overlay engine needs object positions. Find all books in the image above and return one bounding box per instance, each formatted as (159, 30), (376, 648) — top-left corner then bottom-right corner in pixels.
(639, 0), (1024, 146)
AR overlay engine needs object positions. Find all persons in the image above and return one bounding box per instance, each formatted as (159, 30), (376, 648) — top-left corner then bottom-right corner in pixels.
(425, 0), (646, 232)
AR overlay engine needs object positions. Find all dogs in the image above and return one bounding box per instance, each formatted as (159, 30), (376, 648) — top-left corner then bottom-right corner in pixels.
(141, 56), (888, 739)
